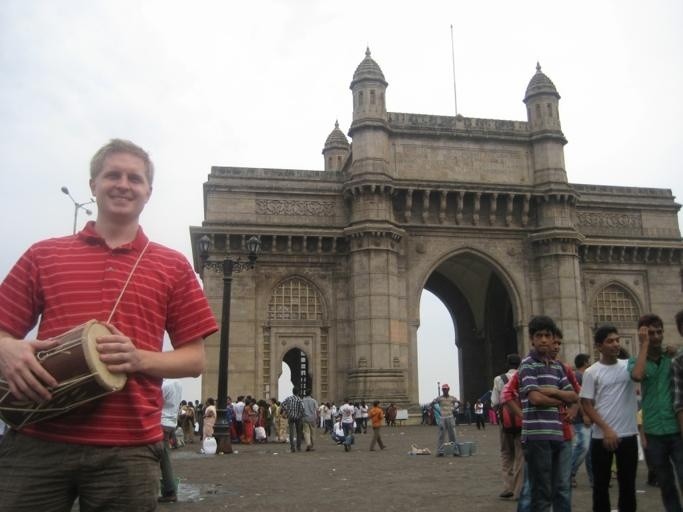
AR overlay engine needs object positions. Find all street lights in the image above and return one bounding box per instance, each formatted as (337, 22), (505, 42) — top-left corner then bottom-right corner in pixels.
(195, 232), (264, 455)
(436, 381), (440, 396)
(59, 185), (96, 234)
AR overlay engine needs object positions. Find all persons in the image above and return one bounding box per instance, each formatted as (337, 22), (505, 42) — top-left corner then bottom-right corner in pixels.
(168, 386), (397, 455)
(0, 136), (221, 512)
(157, 378), (182, 503)
(422, 384), (500, 456)
(489, 315), (683, 512)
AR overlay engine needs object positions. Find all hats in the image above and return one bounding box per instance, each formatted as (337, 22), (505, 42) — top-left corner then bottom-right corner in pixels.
(442, 383), (449, 390)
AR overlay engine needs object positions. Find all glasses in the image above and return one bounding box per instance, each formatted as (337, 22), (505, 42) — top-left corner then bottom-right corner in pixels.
(647, 329), (664, 336)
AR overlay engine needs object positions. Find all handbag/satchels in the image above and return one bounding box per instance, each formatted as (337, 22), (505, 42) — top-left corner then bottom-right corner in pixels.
(254, 426), (266, 440)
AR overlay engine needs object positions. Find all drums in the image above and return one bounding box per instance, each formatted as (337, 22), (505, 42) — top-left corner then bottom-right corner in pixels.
(0, 320), (130, 430)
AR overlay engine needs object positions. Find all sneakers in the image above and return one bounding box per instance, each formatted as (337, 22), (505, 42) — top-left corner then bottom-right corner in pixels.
(157, 494), (178, 502)
(499, 490), (514, 499)
